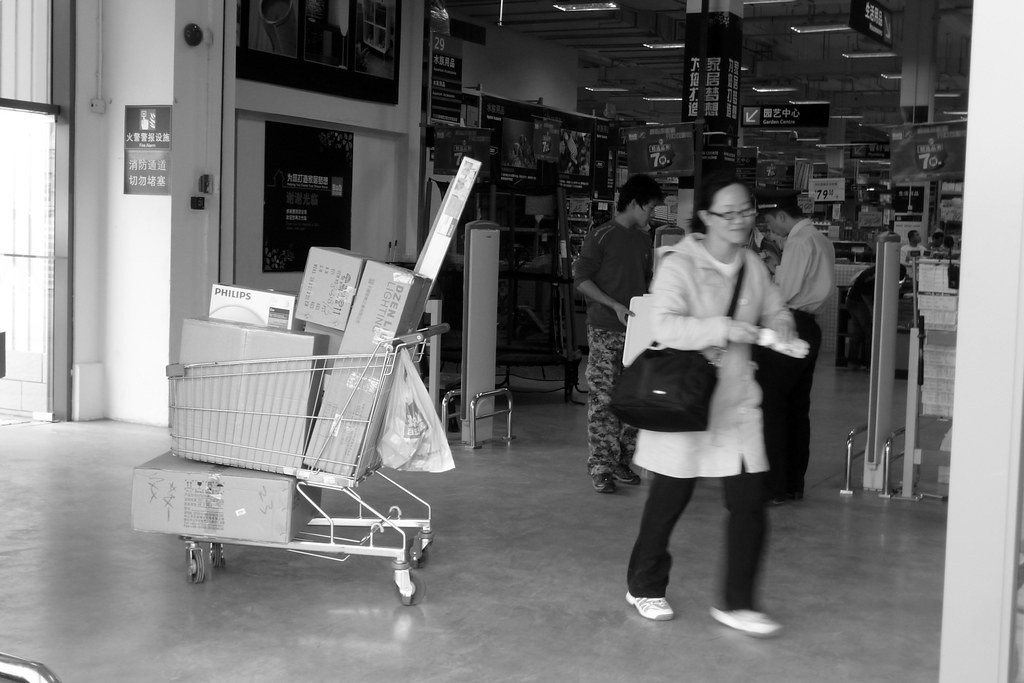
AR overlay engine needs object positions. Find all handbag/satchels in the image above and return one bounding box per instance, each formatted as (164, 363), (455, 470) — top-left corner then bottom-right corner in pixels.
(376, 347), (456, 473)
(609, 265), (744, 432)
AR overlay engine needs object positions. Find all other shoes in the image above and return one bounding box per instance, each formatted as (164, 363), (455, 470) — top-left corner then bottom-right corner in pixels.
(765, 489), (803, 505)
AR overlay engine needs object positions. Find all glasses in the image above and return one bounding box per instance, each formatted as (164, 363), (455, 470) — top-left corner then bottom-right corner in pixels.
(707, 206), (755, 220)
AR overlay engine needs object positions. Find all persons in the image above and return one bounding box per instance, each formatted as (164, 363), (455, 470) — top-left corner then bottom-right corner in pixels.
(574, 174), (954, 637)
(752, 190), (835, 506)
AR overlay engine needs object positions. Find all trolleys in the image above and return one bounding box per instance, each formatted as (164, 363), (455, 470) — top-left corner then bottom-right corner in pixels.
(165, 323), (451, 605)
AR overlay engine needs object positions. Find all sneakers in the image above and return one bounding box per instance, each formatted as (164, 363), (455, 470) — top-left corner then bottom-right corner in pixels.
(612, 464), (641, 484)
(710, 606), (775, 633)
(592, 473), (616, 493)
(626, 590), (673, 621)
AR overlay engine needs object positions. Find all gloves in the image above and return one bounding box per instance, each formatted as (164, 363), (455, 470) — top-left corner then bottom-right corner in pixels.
(756, 328), (810, 360)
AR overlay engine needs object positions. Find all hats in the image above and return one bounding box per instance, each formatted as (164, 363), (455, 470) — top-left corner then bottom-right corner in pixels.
(753, 188), (802, 212)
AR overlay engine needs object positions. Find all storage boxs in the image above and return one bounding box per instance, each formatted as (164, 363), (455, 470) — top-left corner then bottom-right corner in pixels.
(131, 451), (322, 544)
(208, 282), (305, 331)
(303, 260), (432, 477)
(171, 316), (330, 468)
(294, 246), (367, 327)
